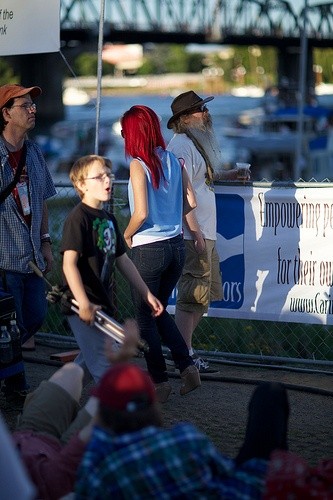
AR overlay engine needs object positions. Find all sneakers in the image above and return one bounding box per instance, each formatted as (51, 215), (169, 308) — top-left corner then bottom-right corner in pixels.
(164, 351), (176, 366)
(175, 353), (220, 375)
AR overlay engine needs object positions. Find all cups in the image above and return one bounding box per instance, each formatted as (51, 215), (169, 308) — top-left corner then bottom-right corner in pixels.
(236, 163), (251, 178)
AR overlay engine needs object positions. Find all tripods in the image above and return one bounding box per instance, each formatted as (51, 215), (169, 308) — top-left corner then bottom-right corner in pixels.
(45, 283), (151, 358)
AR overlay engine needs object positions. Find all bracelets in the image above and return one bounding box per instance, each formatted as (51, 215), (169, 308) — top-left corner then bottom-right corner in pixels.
(41, 233), (50, 242)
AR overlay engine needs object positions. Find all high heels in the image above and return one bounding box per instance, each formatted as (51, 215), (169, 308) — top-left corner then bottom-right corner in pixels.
(178, 365), (201, 394)
(155, 381), (172, 402)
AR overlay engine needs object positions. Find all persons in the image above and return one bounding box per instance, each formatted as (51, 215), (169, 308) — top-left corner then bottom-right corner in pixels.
(0, 84), (58, 409)
(74, 365), (291, 500)
(59, 154), (164, 385)
(120, 105), (202, 403)
(165, 90), (251, 375)
(8, 362), (97, 500)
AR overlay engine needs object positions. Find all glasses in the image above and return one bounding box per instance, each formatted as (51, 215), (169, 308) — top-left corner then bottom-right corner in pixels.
(8, 103), (37, 110)
(81, 172), (115, 182)
(183, 104), (207, 115)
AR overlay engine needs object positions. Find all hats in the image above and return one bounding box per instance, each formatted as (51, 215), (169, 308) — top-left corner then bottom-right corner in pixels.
(0, 83), (42, 108)
(167, 90), (214, 129)
(89, 362), (156, 411)
